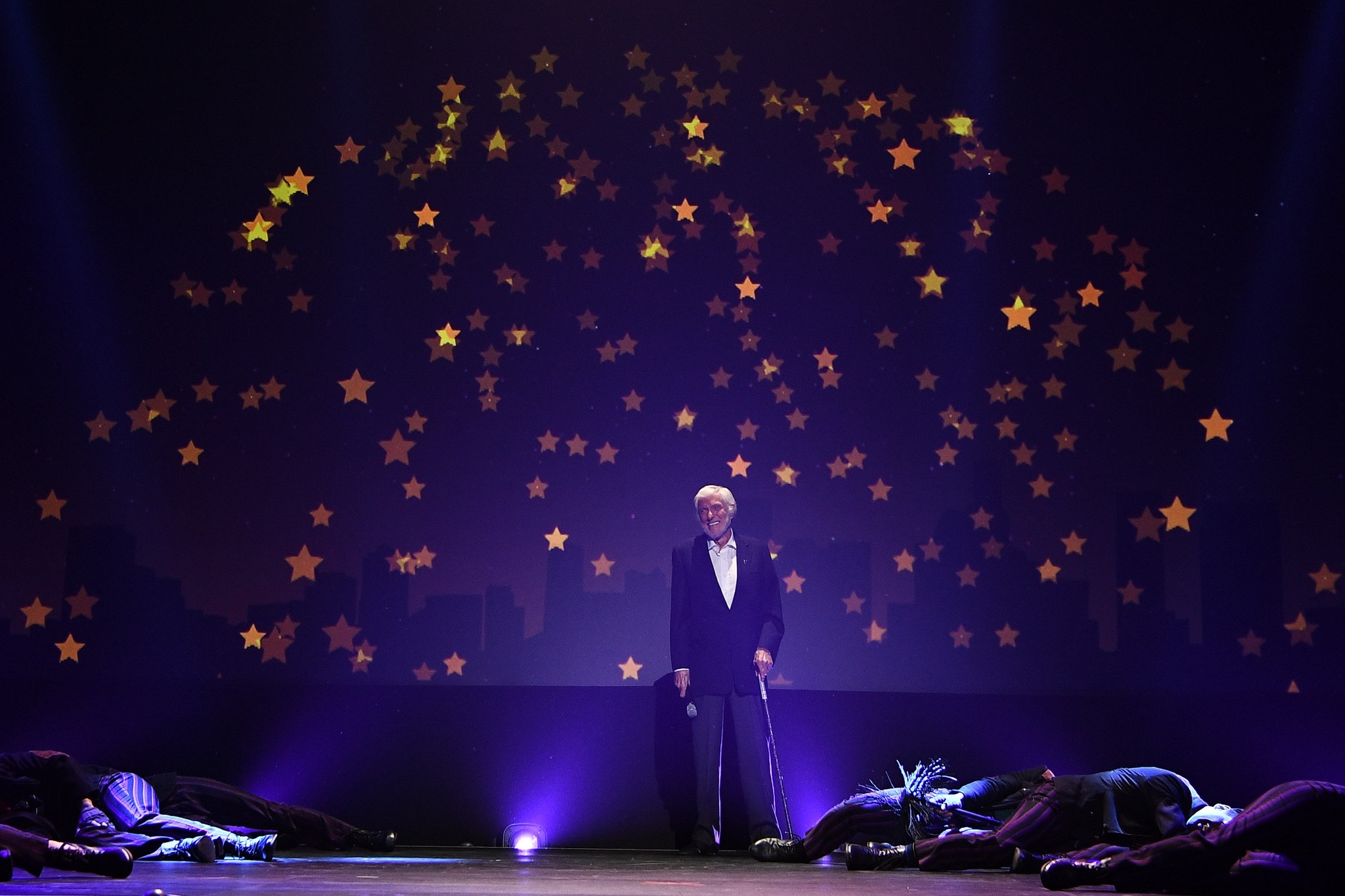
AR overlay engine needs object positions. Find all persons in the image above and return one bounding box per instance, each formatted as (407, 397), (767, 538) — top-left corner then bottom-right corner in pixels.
(1, 824), (134, 883)
(1040, 780), (1344, 895)
(670, 485), (787, 858)
(138, 770), (398, 855)
(747, 767), (1057, 868)
(0, 750), (280, 862)
(845, 768), (1245, 871)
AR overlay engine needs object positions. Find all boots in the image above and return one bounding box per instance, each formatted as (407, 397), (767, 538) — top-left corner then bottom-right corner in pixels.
(225, 832), (278, 862)
(1040, 857), (1113, 891)
(161, 835), (224, 863)
(348, 828), (398, 852)
(49, 839), (134, 879)
(1009, 847), (1071, 873)
(844, 839), (920, 870)
(749, 837), (813, 863)
(0, 849), (13, 882)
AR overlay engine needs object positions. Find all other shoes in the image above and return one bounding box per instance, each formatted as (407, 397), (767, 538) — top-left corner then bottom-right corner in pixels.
(678, 840), (721, 855)
